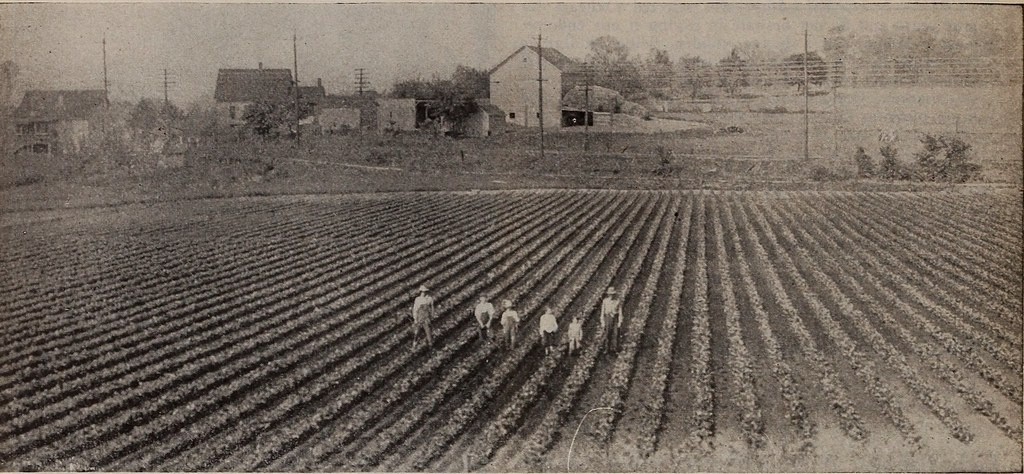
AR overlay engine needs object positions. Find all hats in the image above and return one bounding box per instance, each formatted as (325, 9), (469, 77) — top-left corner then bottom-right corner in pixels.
(607, 287), (616, 294)
(479, 293), (487, 298)
(419, 285), (429, 291)
(503, 300), (515, 308)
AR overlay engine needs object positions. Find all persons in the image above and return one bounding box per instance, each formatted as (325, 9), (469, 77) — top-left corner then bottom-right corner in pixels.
(539, 305), (559, 356)
(413, 284), (436, 353)
(600, 285), (624, 355)
(567, 315), (583, 357)
(500, 298), (520, 352)
(474, 292), (496, 344)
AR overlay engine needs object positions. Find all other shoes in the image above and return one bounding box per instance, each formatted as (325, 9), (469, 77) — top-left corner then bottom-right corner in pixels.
(614, 350), (619, 354)
(603, 350), (608, 355)
